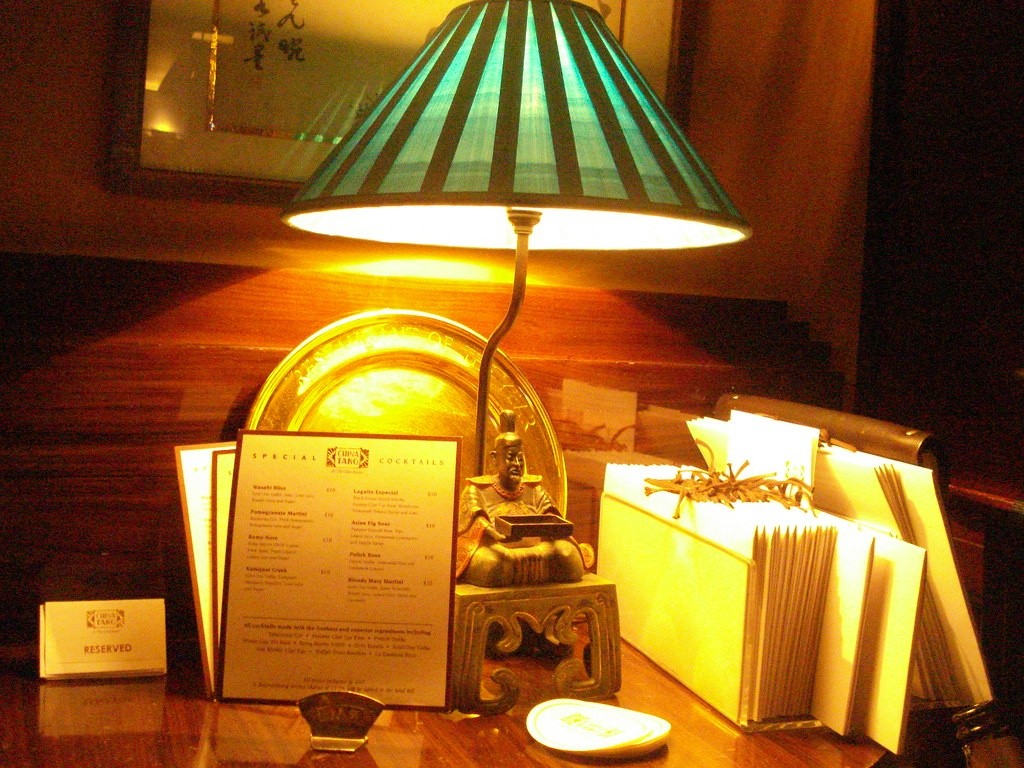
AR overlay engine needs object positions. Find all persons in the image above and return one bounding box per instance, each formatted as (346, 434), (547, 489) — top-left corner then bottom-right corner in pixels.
(456, 409), (588, 586)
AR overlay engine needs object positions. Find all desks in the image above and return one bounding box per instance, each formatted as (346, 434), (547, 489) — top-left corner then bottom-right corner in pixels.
(2, 617), (1023, 768)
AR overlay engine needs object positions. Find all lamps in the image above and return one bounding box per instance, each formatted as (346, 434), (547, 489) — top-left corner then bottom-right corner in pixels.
(281, 0), (755, 478)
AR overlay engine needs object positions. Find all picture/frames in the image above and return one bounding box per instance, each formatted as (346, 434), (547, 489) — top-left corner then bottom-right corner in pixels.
(108, 0), (699, 207)
